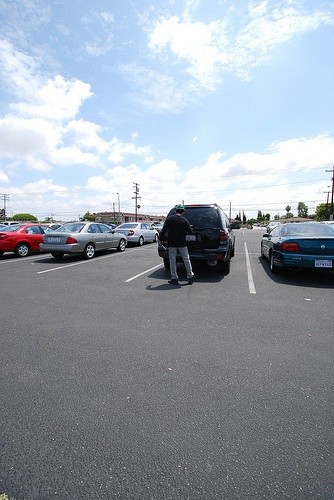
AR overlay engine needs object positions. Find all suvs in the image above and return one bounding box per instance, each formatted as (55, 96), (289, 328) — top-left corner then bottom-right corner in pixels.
(266, 222), (280, 233)
(158, 204), (236, 277)
(0, 222), (20, 230)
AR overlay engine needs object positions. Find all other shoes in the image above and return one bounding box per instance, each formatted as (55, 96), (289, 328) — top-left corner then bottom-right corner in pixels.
(188, 277), (194, 284)
(168, 279), (179, 285)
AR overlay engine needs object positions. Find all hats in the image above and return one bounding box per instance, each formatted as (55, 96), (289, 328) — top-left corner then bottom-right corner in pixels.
(176, 204), (185, 210)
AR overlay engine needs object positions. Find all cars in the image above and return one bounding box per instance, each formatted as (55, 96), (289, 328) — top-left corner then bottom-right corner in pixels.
(115, 222), (159, 246)
(39, 221), (128, 258)
(150, 221), (163, 232)
(0, 224), (56, 257)
(43, 223), (71, 234)
(260, 223), (334, 273)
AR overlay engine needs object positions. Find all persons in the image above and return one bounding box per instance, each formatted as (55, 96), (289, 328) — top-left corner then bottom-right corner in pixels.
(159, 204), (195, 284)
